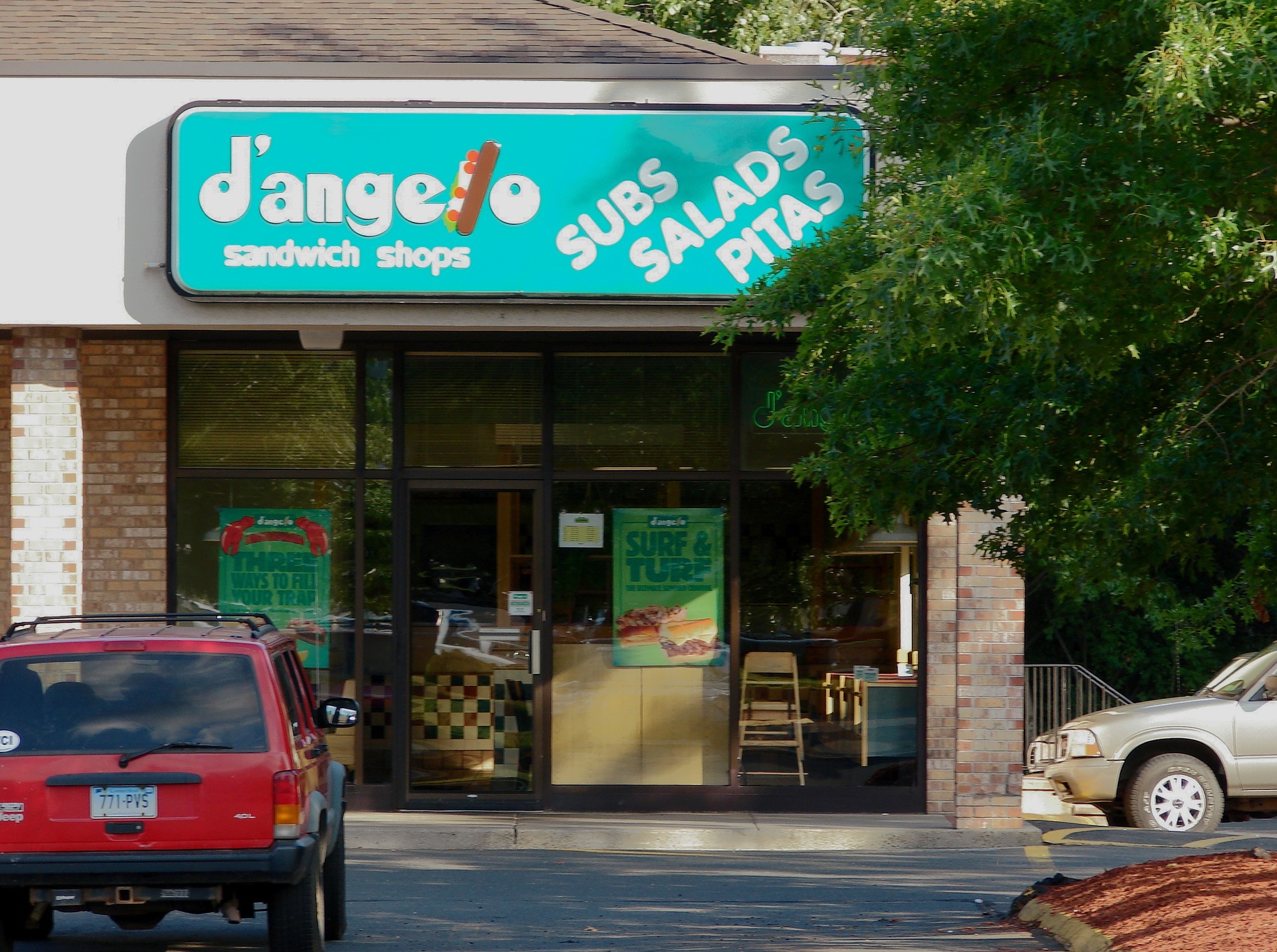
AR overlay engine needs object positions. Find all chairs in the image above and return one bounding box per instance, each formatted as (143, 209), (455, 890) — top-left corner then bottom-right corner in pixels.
(44, 681), (97, 728)
(737, 652), (809, 787)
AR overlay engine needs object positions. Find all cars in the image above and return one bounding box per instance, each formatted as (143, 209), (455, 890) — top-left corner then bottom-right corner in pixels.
(1027, 640), (1277, 832)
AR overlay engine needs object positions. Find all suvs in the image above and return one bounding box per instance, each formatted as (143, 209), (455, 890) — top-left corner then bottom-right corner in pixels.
(1, 609), (361, 952)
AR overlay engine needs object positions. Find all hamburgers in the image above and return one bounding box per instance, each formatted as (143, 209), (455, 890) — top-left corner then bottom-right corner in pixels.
(658, 618), (721, 662)
